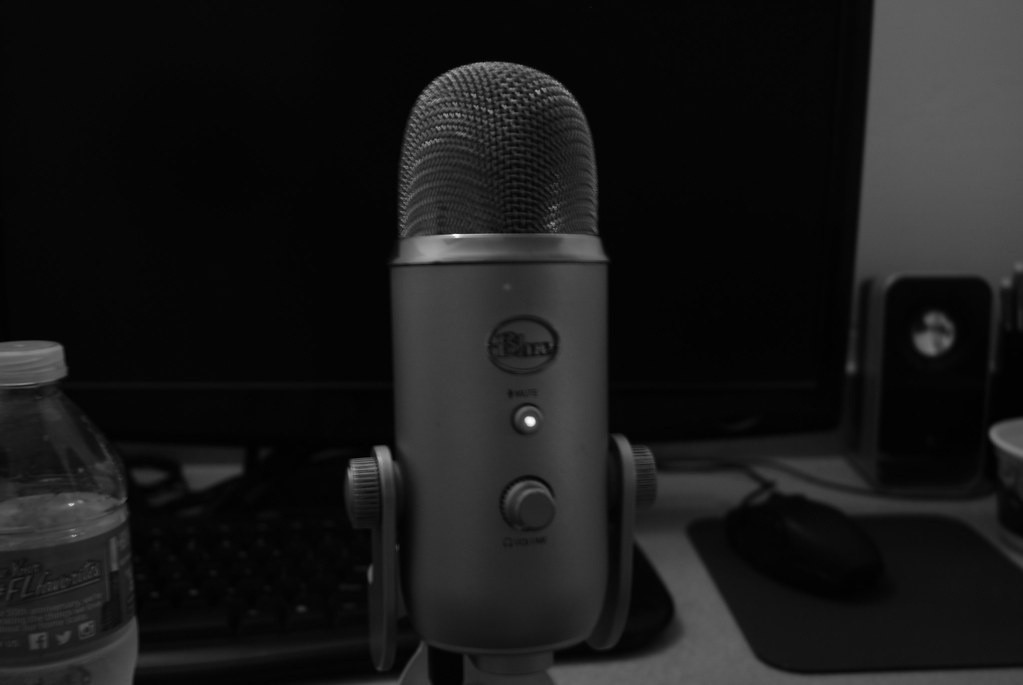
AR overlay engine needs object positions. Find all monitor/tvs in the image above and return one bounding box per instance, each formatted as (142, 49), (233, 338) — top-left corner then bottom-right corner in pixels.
(0, 0), (875, 468)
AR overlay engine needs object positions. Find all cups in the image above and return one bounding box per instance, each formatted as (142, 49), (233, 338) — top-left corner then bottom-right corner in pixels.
(989, 417), (1023, 549)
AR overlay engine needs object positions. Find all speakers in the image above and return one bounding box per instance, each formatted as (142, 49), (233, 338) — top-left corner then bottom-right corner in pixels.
(872, 273), (997, 494)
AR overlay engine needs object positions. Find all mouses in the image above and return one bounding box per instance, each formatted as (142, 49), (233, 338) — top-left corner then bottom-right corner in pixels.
(722, 492), (887, 603)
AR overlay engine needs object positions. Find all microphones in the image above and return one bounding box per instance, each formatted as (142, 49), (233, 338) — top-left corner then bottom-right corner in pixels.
(384, 59), (619, 685)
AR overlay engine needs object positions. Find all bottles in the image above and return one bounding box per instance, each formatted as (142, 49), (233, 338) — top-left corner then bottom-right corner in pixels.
(0, 340), (138, 684)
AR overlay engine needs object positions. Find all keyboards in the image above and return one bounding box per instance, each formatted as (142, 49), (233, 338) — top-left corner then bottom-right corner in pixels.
(121, 501), (675, 659)
